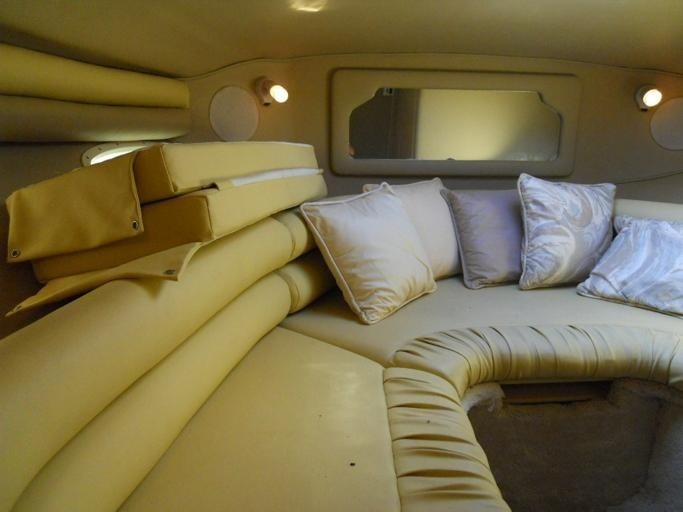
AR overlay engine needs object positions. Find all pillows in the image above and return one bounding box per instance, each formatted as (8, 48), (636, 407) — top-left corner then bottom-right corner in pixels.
(576, 210), (683, 319)
(516, 169), (618, 290)
(300, 181), (438, 326)
(363, 175), (462, 280)
(440, 188), (524, 290)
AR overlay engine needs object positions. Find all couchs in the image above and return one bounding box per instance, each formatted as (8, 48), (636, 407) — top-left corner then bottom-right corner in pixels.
(0, 195), (683, 511)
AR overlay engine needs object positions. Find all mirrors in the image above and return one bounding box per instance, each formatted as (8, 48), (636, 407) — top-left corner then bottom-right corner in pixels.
(331, 69), (585, 177)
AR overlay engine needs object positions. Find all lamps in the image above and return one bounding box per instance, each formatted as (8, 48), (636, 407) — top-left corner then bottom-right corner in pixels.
(255, 76), (289, 105)
(636, 84), (663, 111)
